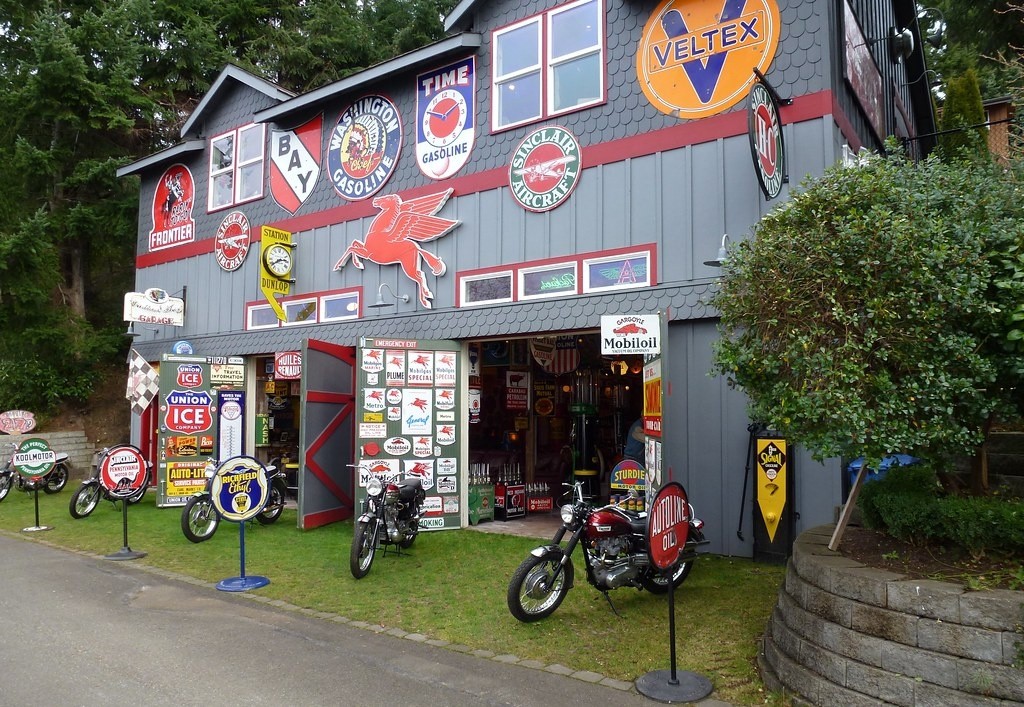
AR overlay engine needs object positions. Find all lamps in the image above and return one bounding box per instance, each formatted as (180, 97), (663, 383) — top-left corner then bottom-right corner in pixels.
(703, 234), (736, 268)
(122, 321), (159, 337)
(868, 6), (944, 59)
(367, 283), (410, 308)
(901, 70), (944, 89)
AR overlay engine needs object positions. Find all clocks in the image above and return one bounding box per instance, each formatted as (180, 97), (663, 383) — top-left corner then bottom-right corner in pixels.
(262, 244), (293, 278)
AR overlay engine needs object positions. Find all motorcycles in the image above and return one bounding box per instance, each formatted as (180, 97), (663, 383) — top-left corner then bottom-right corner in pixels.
(0, 442), (70, 502)
(181, 453), (287, 544)
(69, 447), (153, 519)
(507, 480), (712, 622)
(344, 463), (428, 580)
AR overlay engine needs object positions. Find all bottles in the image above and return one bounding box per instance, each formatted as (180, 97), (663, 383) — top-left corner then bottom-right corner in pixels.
(525, 482), (548, 497)
(497, 463), (522, 486)
(468, 464), (490, 486)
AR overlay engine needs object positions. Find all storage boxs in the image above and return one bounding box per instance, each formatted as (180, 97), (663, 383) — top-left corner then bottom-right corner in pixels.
(494, 484), (526, 521)
(526, 495), (553, 516)
(469, 484), (496, 525)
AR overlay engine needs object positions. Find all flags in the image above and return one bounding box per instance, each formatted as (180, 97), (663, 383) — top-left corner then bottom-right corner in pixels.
(125, 351), (159, 415)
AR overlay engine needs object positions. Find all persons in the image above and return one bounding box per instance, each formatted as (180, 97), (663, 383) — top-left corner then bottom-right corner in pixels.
(625, 410), (644, 463)
